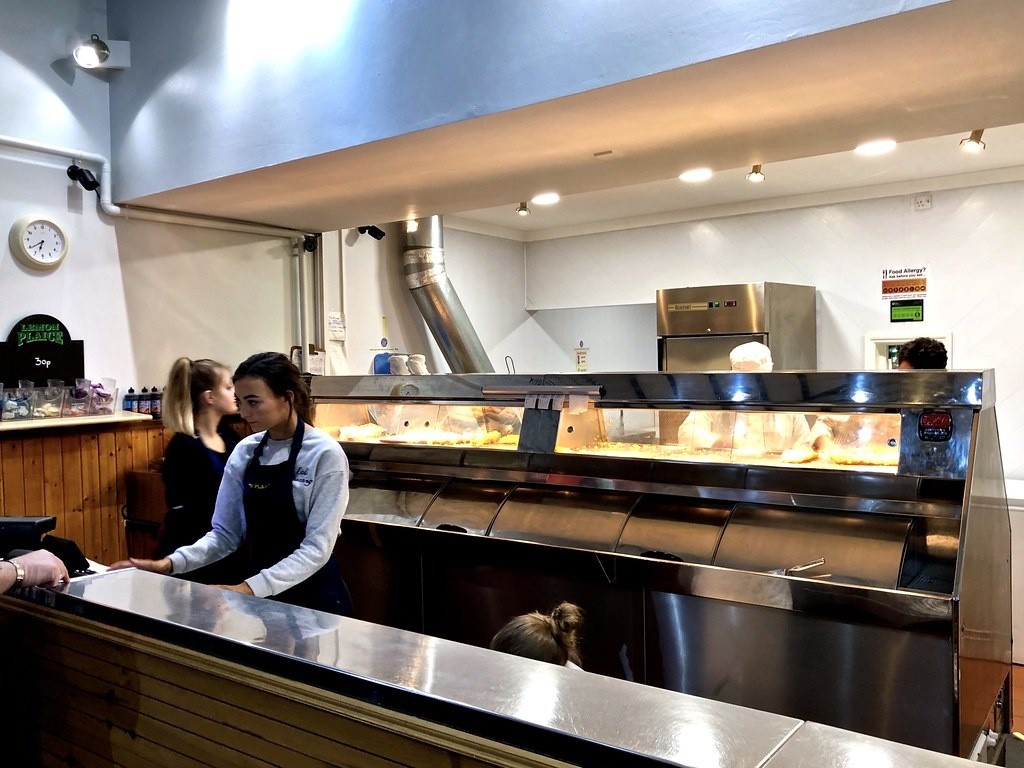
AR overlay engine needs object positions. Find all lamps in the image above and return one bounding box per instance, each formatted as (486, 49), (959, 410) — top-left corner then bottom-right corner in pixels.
(73, 38), (109, 70)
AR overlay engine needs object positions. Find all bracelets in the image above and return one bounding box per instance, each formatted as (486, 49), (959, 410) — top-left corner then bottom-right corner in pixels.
(8, 560), (25, 589)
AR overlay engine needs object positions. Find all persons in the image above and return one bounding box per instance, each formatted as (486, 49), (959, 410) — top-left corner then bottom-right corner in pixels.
(105, 351), (355, 617)
(489, 602), (583, 672)
(678, 336), (947, 467)
(1, 548), (71, 595)
(151, 355), (243, 587)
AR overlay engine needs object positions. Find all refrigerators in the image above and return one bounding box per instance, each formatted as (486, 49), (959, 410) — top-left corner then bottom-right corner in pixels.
(957, 479), (1024, 665)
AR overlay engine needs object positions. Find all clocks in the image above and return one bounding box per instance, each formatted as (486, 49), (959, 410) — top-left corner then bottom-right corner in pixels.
(10, 215), (68, 270)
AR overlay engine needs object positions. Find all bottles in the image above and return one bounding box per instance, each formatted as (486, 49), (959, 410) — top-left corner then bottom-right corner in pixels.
(149, 386), (162, 419)
(123, 386), (139, 412)
(138, 387), (152, 415)
